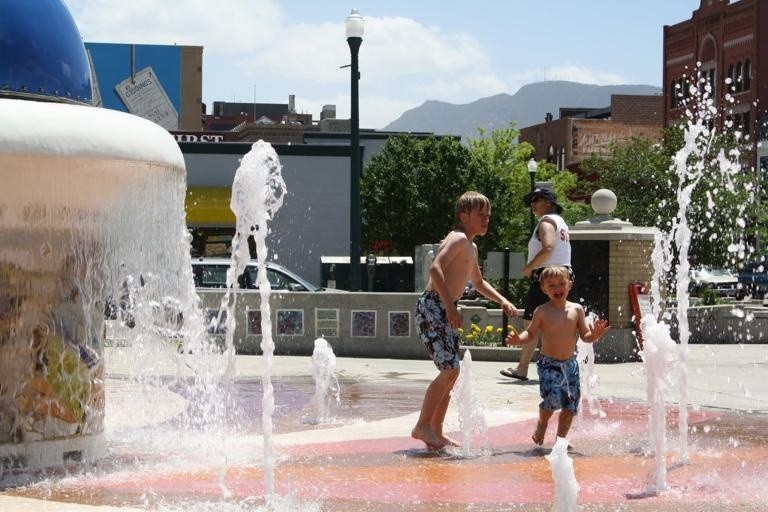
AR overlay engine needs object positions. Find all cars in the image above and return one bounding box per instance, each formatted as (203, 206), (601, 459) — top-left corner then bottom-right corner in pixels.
(737, 253), (768, 299)
(667, 262), (746, 301)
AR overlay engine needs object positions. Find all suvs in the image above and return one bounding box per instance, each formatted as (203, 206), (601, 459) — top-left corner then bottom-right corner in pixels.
(189, 255), (352, 336)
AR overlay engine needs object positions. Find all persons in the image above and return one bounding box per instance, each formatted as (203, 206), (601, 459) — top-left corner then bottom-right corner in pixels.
(499, 184), (579, 379)
(405, 190), (519, 454)
(500, 264), (614, 455)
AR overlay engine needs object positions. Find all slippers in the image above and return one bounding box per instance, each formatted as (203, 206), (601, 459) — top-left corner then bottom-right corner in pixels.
(499, 366), (530, 382)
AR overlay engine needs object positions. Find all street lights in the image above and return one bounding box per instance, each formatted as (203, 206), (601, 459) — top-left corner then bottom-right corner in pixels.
(365, 253), (377, 291)
(525, 158), (537, 237)
(341, 7), (365, 294)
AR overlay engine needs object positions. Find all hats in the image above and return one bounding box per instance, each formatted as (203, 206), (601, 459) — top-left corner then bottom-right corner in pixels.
(522, 186), (565, 216)
